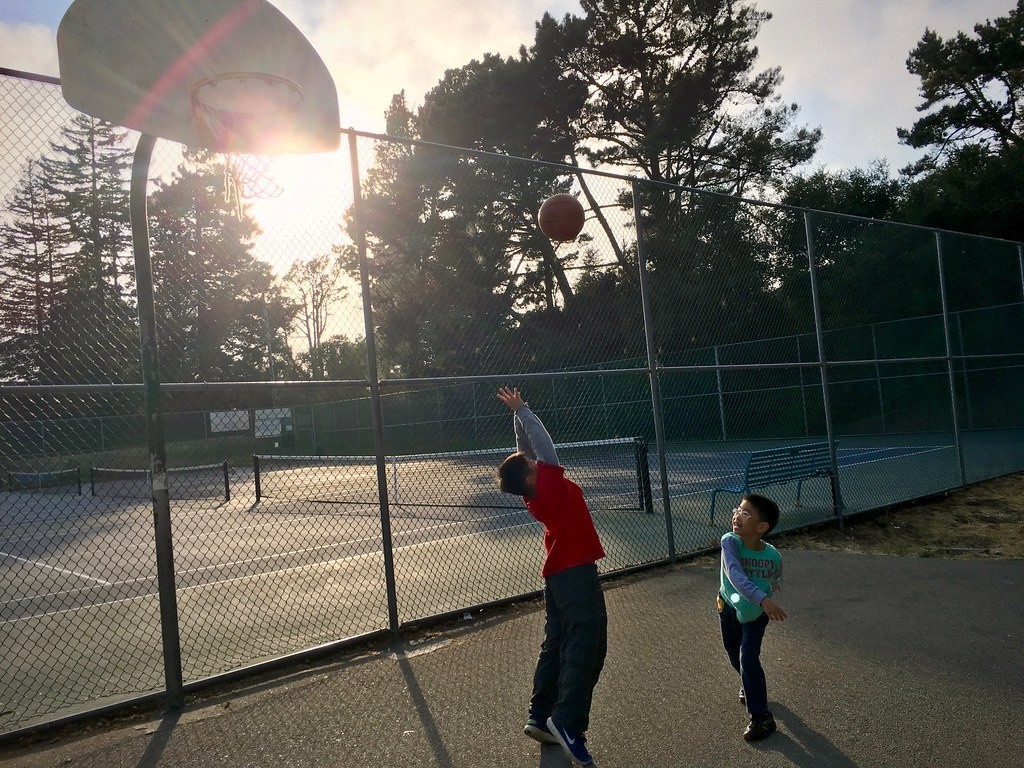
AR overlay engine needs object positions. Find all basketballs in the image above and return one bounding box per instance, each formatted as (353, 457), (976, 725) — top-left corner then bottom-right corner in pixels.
(537, 194), (585, 241)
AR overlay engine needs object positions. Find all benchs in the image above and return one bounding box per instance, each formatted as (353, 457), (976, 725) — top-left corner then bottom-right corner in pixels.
(706, 440), (846, 526)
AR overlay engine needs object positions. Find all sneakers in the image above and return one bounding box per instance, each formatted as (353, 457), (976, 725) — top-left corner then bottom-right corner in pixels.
(739, 686), (746, 701)
(547, 717), (593, 764)
(743, 711), (777, 741)
(524, 716), (560, 744)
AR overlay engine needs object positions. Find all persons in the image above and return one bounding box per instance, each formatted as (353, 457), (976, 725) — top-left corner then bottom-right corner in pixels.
(717, 494), (788, 741)
(496, 385), (608, 765)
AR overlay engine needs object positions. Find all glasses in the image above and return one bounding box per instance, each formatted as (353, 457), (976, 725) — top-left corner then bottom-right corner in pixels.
(732, 508), (761, 523)
